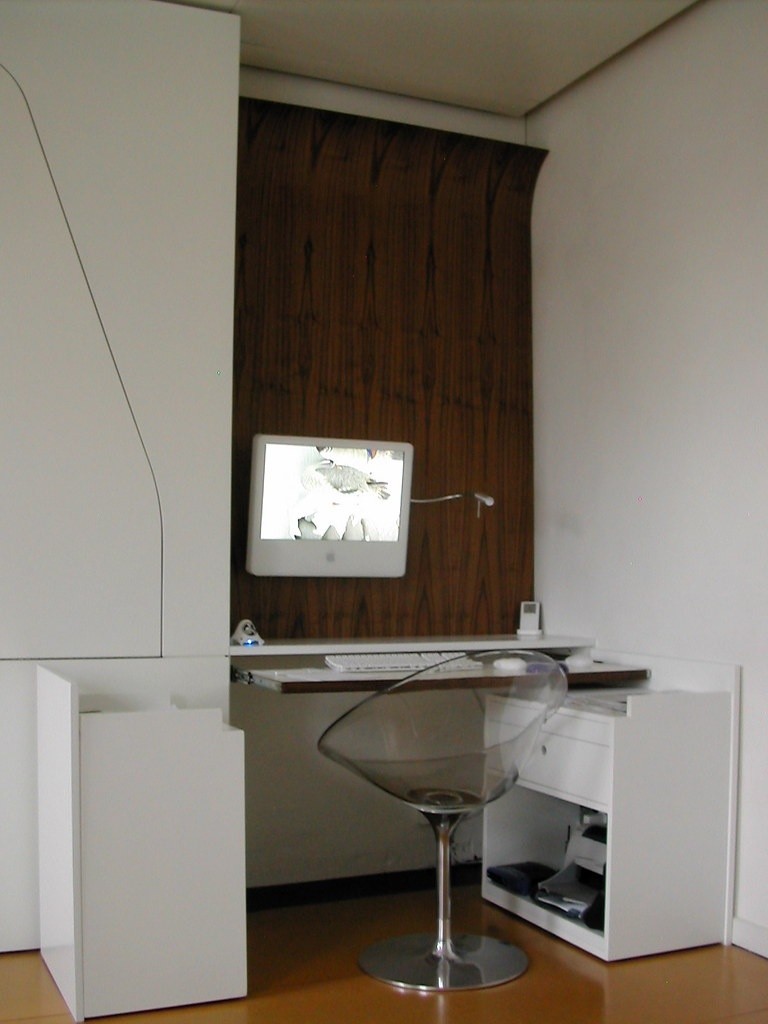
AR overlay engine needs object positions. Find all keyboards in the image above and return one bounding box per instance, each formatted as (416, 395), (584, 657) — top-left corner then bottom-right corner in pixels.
(324, 652), (482, 673)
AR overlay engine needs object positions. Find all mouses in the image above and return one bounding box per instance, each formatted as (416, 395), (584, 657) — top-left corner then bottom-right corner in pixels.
(493, 657), (527, 670)
(565, 654), (593, 666)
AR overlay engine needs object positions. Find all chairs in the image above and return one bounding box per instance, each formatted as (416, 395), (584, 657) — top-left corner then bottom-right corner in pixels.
(318, 649), (568, 992)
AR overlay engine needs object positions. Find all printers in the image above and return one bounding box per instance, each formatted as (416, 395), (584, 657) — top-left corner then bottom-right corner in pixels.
(534, 822), (607, 930)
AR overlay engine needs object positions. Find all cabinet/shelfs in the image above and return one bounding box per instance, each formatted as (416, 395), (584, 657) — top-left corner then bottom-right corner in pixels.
(36, 665), (246, 1024)
(483, 650), (742, 962)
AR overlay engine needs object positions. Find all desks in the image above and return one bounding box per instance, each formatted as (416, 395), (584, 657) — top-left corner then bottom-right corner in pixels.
(231, 635), (651, 695)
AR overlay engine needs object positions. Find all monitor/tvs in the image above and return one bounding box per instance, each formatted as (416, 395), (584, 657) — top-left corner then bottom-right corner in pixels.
(245, 434), (413, 578)
